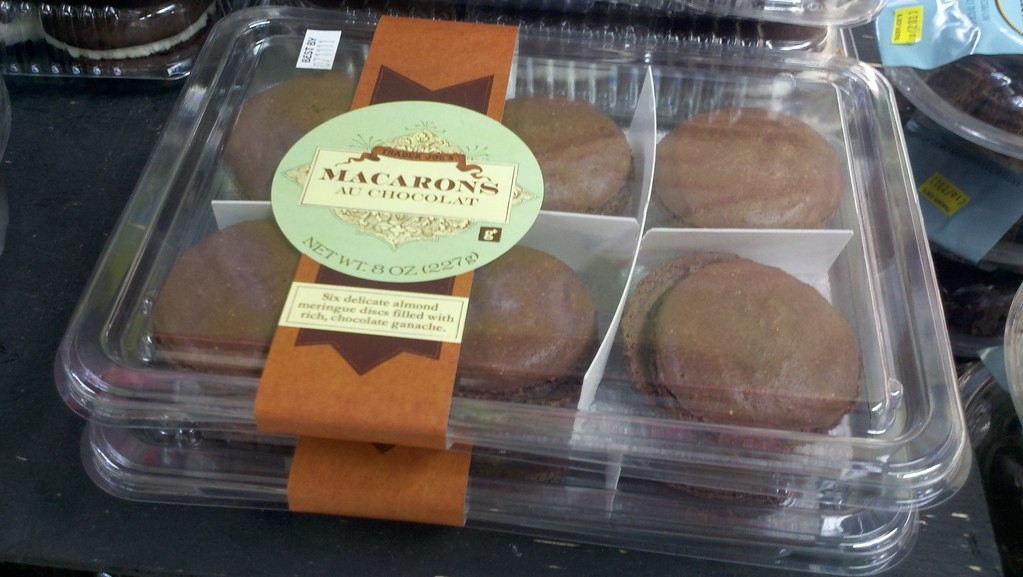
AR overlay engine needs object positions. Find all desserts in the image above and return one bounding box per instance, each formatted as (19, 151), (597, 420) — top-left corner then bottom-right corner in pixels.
(0, 0), (224, 61)
(154, 72), (870, 456)
